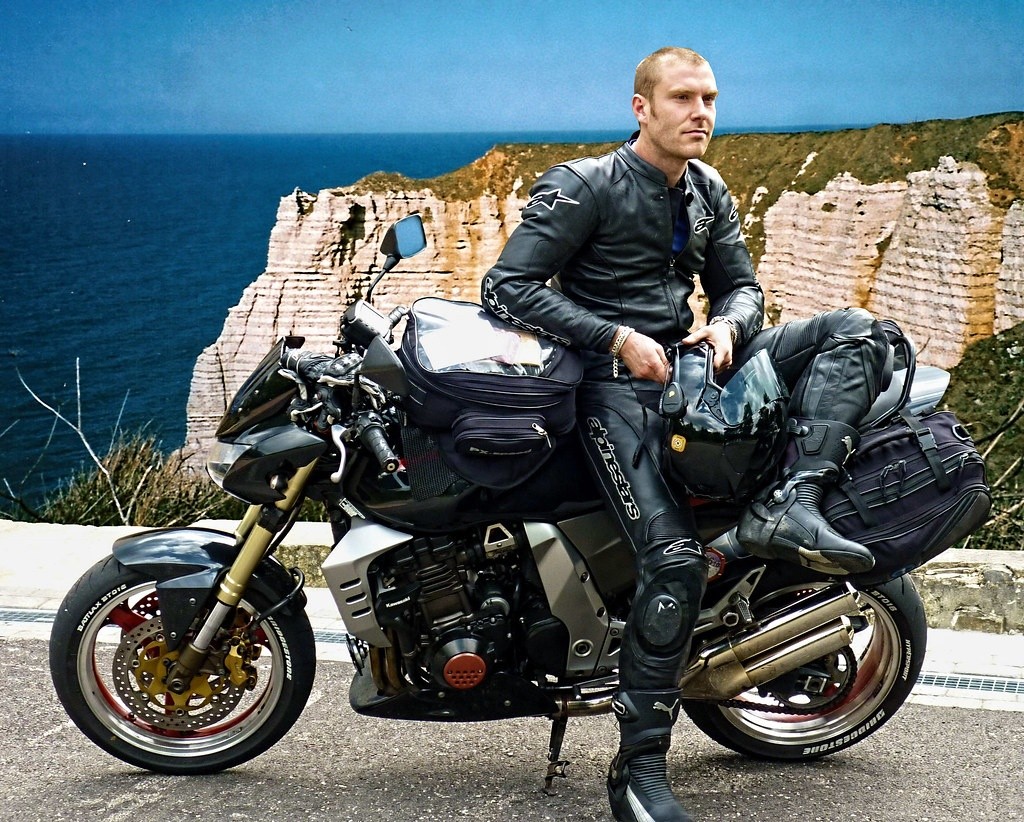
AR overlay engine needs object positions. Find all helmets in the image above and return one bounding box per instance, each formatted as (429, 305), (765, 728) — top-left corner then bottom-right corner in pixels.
(658, 338), (791, 512)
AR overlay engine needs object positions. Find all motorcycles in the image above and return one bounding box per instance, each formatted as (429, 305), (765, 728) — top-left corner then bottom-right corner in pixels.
(49, 214), (993, 797)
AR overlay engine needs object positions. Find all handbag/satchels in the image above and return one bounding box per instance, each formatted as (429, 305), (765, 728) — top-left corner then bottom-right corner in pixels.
(400, 297), (585, 489)
(820, 408), (991, 589)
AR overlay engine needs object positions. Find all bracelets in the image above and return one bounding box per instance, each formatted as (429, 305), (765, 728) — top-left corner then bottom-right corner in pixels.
(710, 315), (737, 344)
(610, 325), (636, 357)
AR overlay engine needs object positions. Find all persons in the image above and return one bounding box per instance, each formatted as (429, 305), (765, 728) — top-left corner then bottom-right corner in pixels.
(480, 45), (895, 822)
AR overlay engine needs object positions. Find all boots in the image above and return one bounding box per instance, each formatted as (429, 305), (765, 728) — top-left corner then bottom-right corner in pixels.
(736, 416), (876, 574)
(607, 688), (693, 822)
